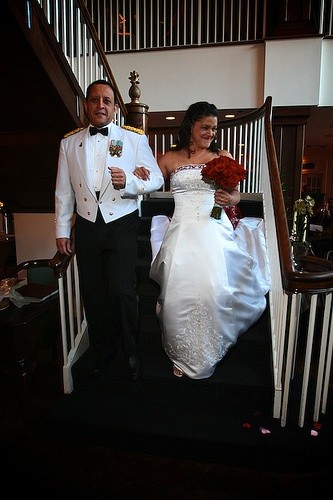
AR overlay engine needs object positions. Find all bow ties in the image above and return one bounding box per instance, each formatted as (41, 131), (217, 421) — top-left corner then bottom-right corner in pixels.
(89, 127), (108, 137)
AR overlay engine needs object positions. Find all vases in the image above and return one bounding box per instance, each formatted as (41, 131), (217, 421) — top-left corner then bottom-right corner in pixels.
(295, 246), (309, 257)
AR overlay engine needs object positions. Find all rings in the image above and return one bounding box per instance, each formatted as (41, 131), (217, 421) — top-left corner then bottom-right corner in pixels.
(220, 193), (223, 196)
(218, 197), (221, 201)
(115, 173), (119, 178)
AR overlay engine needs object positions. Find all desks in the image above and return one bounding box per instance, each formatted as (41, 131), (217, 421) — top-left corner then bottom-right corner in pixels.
(0, 284), (59, 432)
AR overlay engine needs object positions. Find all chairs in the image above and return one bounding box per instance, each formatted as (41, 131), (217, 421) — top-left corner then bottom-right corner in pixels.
(17, 259), (75, 362)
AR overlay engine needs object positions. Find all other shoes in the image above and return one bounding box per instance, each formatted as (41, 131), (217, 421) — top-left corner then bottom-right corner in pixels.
(128, 358), (141, 381)
(89, 362), (106, 378)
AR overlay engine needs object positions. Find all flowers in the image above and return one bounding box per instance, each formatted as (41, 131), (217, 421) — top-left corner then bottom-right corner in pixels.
(201, 155), (248, 220)
(290, 195), (315, 257)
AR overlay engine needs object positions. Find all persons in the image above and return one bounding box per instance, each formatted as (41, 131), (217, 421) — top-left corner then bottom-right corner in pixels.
(133, 101), (241, 379)
(55, 79), (165, 380)
